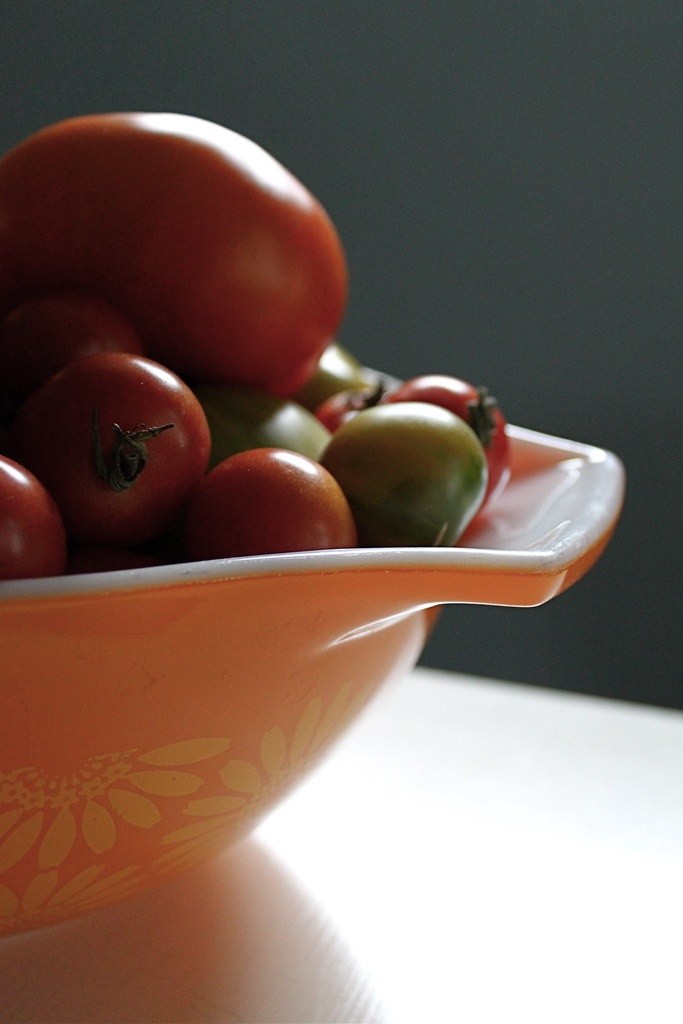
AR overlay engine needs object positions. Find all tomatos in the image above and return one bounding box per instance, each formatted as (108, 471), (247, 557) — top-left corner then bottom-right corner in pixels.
(0, 110), (512, 579)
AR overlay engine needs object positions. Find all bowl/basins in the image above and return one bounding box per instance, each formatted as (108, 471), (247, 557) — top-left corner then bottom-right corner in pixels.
(0, 370), (626, 939)
